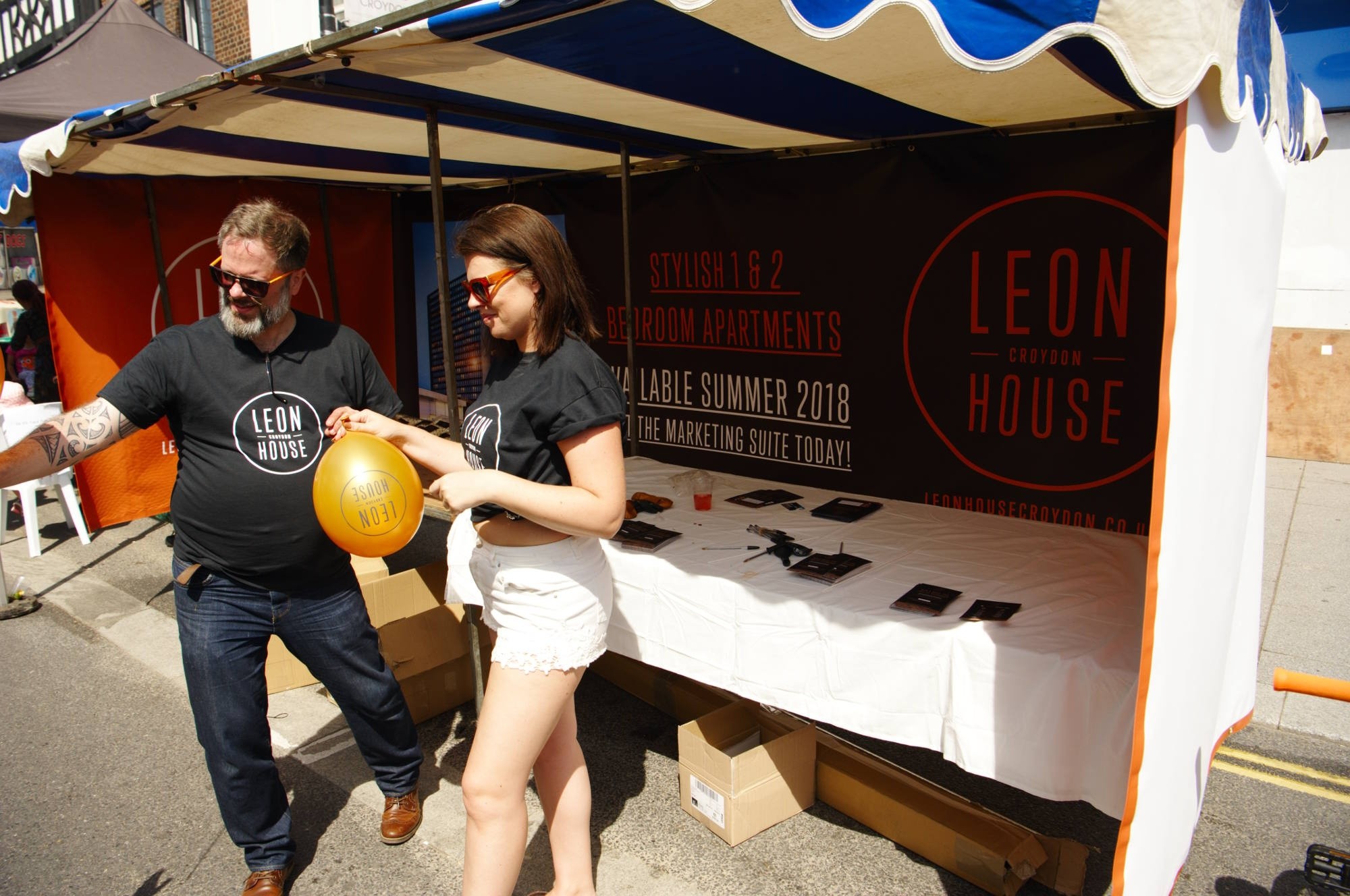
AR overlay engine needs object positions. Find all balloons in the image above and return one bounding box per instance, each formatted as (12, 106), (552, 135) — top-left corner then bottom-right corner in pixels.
(311, 416), (425, 558)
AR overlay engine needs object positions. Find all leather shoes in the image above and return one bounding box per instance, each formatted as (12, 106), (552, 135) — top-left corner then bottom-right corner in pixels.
(380, 787), (422, 844)
(243, 862), (297, 896)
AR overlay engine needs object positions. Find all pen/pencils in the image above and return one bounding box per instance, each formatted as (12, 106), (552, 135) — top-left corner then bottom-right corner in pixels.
(839, 542), (843, 553)
(746, 524), (795, 544)
(702, 545), (760, 550)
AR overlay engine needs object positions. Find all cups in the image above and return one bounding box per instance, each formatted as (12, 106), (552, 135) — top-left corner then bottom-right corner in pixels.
(693, 476), (713, 511)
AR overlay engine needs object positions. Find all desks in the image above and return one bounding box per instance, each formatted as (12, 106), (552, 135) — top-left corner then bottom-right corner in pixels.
(382, 450), (1151, 823)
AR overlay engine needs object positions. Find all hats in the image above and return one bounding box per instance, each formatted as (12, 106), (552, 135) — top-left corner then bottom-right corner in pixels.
(12, 280), (46, 303)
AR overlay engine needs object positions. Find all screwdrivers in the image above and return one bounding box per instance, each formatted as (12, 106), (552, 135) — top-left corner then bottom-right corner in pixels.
(743, 542), (786, 563)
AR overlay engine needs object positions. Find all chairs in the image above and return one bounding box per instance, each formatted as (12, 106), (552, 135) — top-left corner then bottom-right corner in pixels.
(0, 402), (91, 557)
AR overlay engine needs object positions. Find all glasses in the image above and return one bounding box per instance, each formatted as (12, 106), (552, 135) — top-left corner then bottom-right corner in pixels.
(208, 255), (291, 299)
(461, 262), (529, 305)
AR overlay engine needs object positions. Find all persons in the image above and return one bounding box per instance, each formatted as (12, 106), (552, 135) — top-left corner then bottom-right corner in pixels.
(7, 278), (59, 404)
(0, 195), (430, 896)
(325, 203), (628, 896)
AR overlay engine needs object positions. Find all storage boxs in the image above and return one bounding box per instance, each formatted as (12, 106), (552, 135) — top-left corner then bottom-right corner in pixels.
(588, 650), (1090, 896)
(264, 553), (497, 727)
